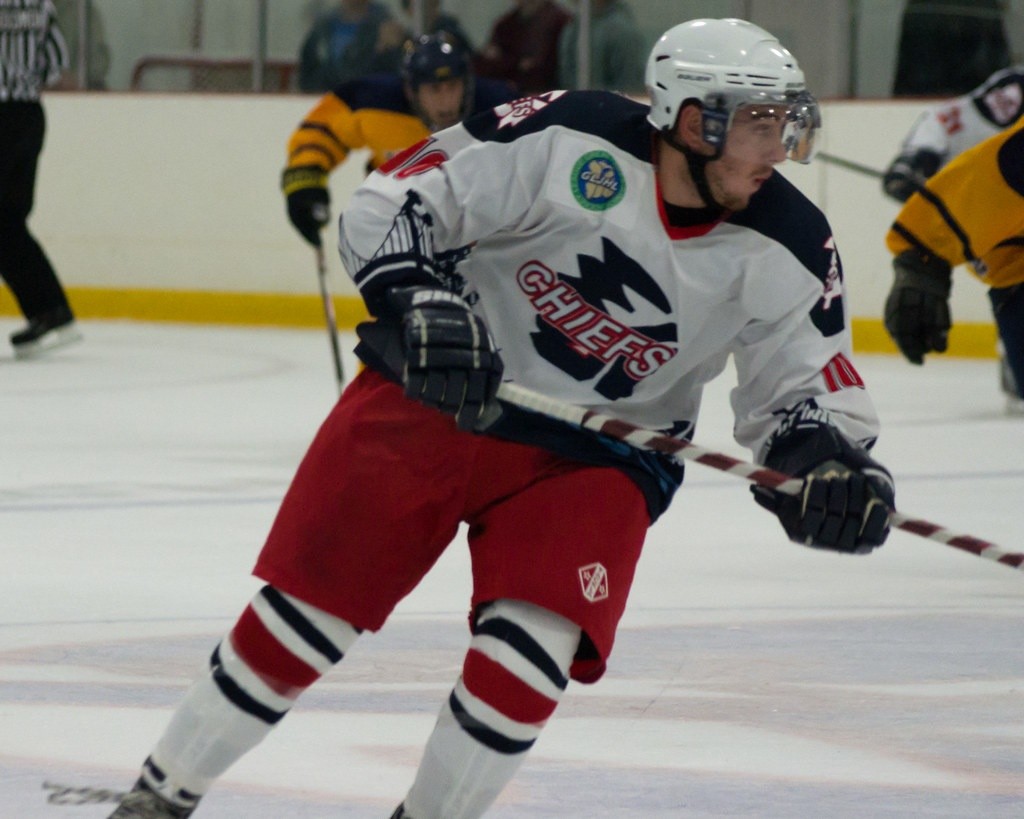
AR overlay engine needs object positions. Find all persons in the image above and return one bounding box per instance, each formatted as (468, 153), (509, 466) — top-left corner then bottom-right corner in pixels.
(108, 20), (897, 819)
(278, 27), (532, 242)
(887, 60), (1024, 197)
(885, 104), (1024, 401)
(0, 2), (87, 353)
(285, 0), (650, 103)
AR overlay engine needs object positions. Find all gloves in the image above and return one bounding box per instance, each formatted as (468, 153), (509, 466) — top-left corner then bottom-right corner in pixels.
(885, 250), (952, 365)
(748, 431), (897, 554)
(373, 286), (505, 433)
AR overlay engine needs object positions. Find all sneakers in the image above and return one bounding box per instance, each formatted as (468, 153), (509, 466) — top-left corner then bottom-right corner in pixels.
(9, 315), (82, 357)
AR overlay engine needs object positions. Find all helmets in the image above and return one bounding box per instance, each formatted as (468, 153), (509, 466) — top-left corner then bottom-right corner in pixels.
(397, 30), (481, 81)
(640, 16), (807, 160)
